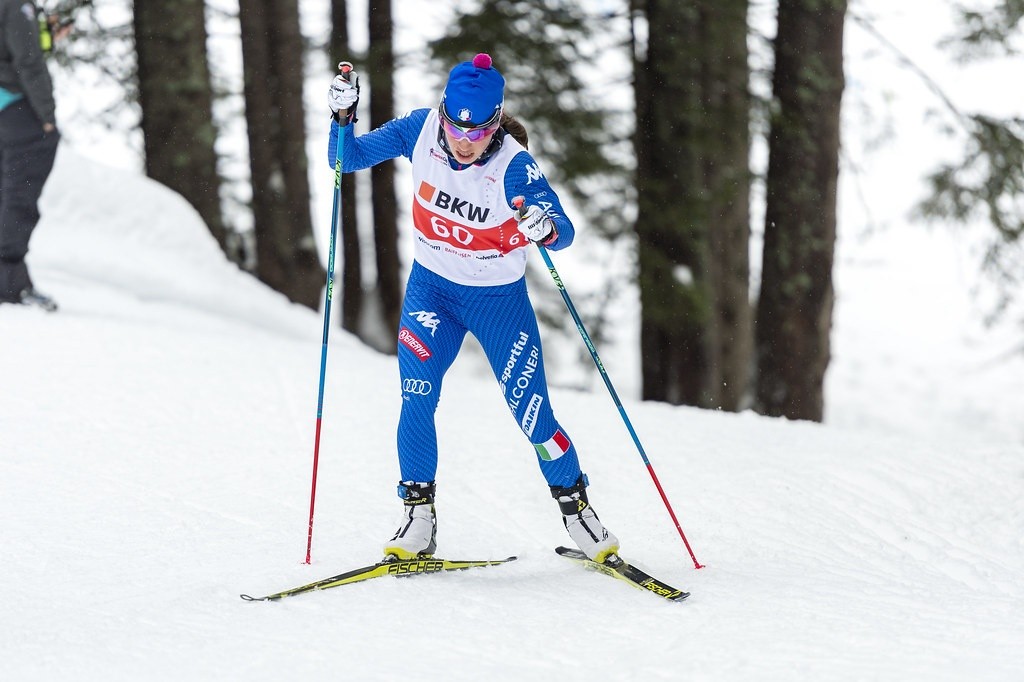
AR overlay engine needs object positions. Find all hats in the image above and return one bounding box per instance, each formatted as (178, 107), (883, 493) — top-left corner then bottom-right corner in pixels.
(444, 53), (506, 128)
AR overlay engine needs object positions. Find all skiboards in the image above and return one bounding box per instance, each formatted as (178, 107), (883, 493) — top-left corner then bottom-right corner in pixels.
(240, 543), (691, 603)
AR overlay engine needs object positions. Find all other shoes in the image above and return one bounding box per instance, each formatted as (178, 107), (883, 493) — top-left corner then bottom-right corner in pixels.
(15, 289), (56, 311)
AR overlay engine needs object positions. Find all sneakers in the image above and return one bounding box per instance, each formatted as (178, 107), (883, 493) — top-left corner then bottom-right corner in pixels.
(383, 504), (437, 559)
(557, 493), (619, 563)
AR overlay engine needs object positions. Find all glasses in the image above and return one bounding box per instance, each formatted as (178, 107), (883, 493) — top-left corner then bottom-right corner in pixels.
(438, 102), (501, 143)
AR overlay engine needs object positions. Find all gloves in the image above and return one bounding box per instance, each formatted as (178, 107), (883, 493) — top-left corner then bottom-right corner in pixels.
(328, 72), (360, 119)
(513, 205), (552, 242)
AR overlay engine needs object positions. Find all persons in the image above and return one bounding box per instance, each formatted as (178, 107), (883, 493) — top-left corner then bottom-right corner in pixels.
(327, 52), (619, 564)
(0, 0), (70, 310)
